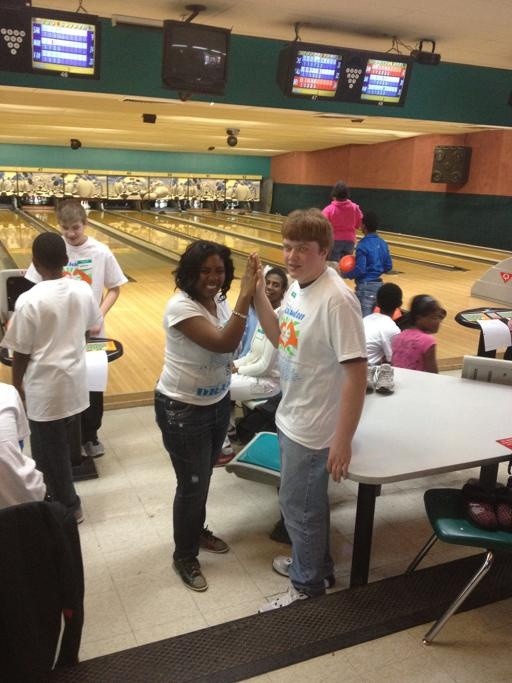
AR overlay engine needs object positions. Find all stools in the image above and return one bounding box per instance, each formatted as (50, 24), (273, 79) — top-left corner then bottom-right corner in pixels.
(226, 431), (292, 544)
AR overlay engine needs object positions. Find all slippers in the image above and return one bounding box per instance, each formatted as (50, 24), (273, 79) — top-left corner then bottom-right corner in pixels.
(494, 486), (512, 527)
(462, 482), (497, 530)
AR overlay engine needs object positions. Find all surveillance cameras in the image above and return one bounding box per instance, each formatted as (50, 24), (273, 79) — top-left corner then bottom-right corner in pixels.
(70, 139), (81, 149)
(226, 128), (241, 146)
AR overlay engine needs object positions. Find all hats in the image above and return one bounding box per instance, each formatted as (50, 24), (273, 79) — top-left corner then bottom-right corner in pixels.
(411, 295), (447, 320)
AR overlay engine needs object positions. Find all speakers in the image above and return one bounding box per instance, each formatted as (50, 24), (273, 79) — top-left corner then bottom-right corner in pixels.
(142, 113), (156, 122)
(411, 50), (441, 65)
(431, 144), (472, 187)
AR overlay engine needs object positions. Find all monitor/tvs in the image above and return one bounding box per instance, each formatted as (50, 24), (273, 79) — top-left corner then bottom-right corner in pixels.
(275, 41), (347, 102)
(161, 19), (232, 97)
(351, 52), (413, 102)
(31, 12), (102, 80)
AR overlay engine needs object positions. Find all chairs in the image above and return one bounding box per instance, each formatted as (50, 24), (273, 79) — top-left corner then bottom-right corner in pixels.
(405, 487), (512, 645)
(462, 356), (512, 384)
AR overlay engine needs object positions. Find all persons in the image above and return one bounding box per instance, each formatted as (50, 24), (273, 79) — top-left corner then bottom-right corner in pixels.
(212, 268), (288, 467)
(335, 212), (392, 317)
(24, 199), (129, 457)
(252, 208), (369, 613)
(1, 232), (104, 525)
(0, 384), (47, 510)
(154, 240), (256, 591)
(391, 294), (447, 374)
(362, 283), (404, 366)
(321, 181), (364, 262)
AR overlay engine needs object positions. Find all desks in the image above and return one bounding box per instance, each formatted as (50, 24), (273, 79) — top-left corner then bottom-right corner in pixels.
(342, 366), (512, 588)
(455, 306), (512, 358)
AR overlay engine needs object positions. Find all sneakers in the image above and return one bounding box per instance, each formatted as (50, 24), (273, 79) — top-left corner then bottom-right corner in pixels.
(173, 557), (208, 591)
(366, 365), (377, 392)
(258, 582), (309, 613)
(73, 440), (105, 522)
(375, 362), (394, 393)
(272, 555), (335, 588)
(199, 523), (229, 553)
(213, 444), (235, 466)
